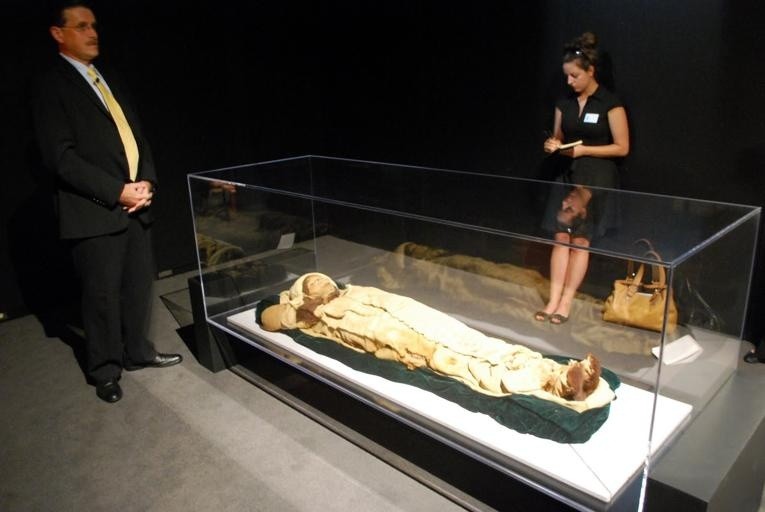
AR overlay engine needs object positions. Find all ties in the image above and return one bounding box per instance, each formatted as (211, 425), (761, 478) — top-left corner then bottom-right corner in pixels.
(86, 68), (139, 183)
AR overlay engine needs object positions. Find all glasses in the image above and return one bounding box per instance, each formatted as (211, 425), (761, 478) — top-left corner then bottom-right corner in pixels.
(561, 46), (591, 62)
(62, 22), (98, 32)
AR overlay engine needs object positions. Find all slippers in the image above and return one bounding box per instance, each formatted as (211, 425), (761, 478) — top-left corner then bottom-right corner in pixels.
(534, 312), (568, 325)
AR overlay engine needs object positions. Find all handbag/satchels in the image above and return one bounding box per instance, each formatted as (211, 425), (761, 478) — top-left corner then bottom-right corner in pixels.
(601, 279), (678, 333)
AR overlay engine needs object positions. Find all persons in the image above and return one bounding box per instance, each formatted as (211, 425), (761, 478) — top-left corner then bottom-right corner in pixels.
(288, 270), (603, 406)
(30, 0), (183, 405)
(533, 29), (631, 327)
(744, 337), (765, 366)
(553, 183), (601, 233)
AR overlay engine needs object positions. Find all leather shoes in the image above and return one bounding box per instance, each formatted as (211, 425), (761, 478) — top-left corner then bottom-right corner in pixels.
(124, 352), (182, 371)
(96, 377), (122, 403)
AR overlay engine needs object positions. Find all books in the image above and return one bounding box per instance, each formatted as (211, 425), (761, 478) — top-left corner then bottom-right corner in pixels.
(558, 138), (583, 150)
